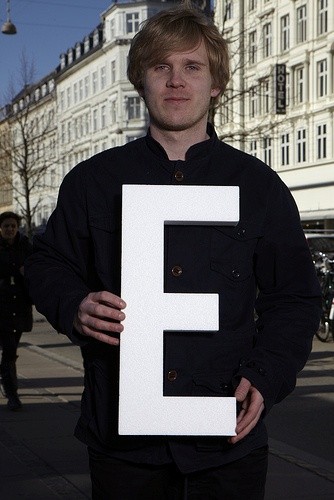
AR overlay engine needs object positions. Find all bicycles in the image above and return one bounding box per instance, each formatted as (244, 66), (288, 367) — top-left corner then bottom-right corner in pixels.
(309, 252), (334, 341)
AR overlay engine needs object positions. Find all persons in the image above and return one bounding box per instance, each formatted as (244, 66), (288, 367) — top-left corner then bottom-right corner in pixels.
(312, 253), (334, 343)
(0, 212), (35, 411)
(35, 9), (323, 500)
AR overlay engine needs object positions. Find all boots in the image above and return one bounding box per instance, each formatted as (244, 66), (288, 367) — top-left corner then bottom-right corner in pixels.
(0, 349), (23, 412)
